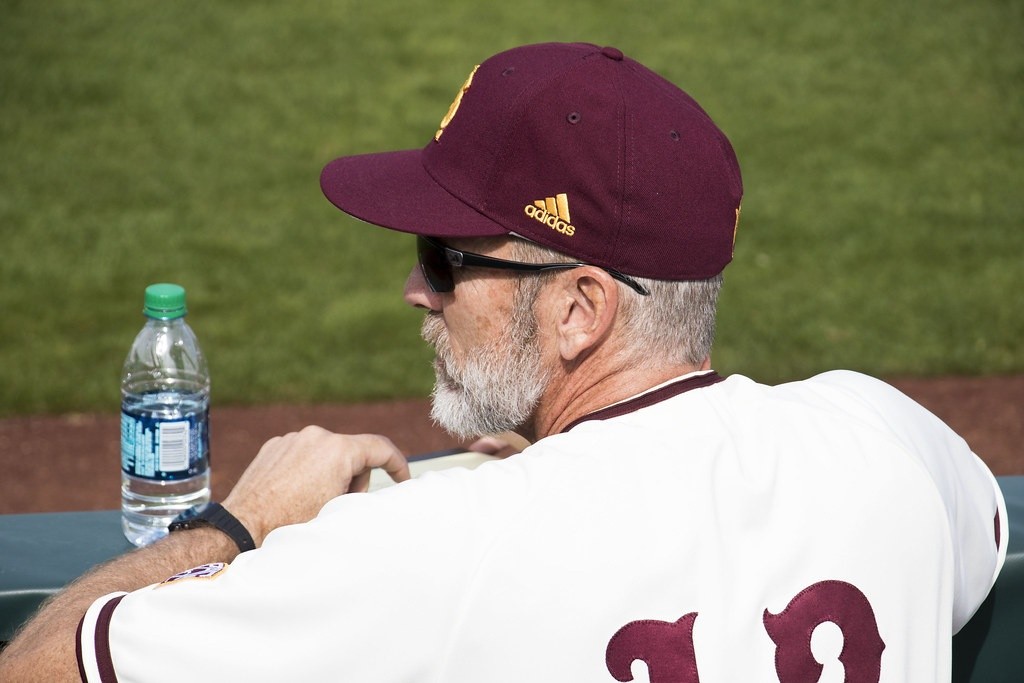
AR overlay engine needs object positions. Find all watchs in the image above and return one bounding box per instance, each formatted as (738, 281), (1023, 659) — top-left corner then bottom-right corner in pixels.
(168, 503), (256, 554)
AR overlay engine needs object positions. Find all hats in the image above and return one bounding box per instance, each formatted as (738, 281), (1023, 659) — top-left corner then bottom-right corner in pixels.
(320, 42), (743, 281)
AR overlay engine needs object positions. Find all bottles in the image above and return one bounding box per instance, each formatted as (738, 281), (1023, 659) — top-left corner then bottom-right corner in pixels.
(120, 282), (212, 547)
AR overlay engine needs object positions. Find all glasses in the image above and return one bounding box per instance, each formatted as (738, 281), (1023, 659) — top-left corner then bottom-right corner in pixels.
(417, 235), (650, 296)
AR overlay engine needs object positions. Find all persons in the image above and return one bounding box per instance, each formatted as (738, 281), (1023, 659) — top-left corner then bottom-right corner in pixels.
(0, 42), (1009, 683)
